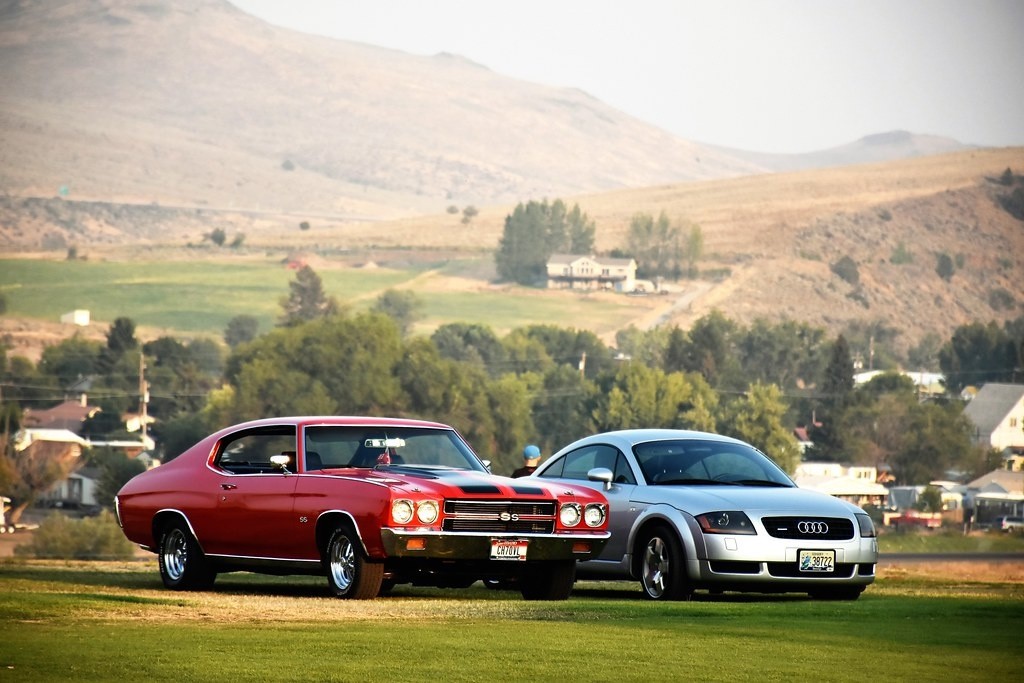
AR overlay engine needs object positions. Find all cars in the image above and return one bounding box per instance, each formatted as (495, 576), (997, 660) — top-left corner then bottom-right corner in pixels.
(992, 514), (1024, 533)
(515, 428), (879, 603)
(107, 414), (614, 602)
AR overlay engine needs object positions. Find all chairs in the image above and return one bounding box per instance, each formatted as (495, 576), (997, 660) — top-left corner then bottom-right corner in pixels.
(274, 451), (322, 474)
(356, 453), (404, 468)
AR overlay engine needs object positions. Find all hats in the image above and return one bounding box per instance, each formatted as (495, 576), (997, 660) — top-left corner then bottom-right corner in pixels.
(524, 445), (539, 459)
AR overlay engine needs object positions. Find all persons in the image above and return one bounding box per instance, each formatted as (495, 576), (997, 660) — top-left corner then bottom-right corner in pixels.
(511, 445), (541, 479)
